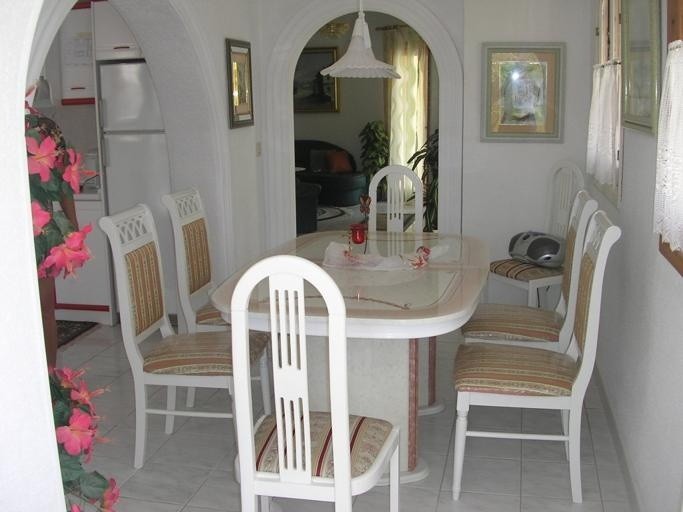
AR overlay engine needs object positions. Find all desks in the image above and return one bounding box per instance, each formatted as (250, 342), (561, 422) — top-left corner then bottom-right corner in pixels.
(210, 231), (492, 486)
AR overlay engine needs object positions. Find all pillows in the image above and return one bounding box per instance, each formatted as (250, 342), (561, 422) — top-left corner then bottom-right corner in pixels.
(326, 151), (353, 173)
(311, 149), (335, 172)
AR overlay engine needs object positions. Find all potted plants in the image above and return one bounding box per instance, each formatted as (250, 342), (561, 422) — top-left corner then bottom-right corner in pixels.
(359, 118), (386, 202)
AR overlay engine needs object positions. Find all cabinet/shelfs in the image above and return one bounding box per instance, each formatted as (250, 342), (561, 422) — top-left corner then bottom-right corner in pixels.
(52, 201), (109, 312)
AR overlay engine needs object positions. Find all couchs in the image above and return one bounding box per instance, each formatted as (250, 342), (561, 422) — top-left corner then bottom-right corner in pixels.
(295, 138), (367, 206)
(297, 183), (321, 238)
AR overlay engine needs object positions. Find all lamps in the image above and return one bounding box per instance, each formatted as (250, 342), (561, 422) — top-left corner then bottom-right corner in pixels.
(321, 2), (400, 80)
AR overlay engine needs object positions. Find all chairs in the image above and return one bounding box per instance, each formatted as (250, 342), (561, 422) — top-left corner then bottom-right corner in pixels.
(99, 203), (272, 469)
(453, 210), (621, 504)
(461, 190), (598, 461)
(161, 186), (273, 408)
(368, 164), (423, 232)
(481, 161), (585, 308)
(231, 255), (401, 512)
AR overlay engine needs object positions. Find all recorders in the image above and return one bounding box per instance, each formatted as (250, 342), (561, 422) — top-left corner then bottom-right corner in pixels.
(508, 232), (567, 267)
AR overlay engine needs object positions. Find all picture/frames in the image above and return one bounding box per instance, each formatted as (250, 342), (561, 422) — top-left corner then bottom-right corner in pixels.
(621, 1), (661, 138)
(294, 46), (340, 112)
(480, 40), (566, 143)
(225, 37), (254, 129)
